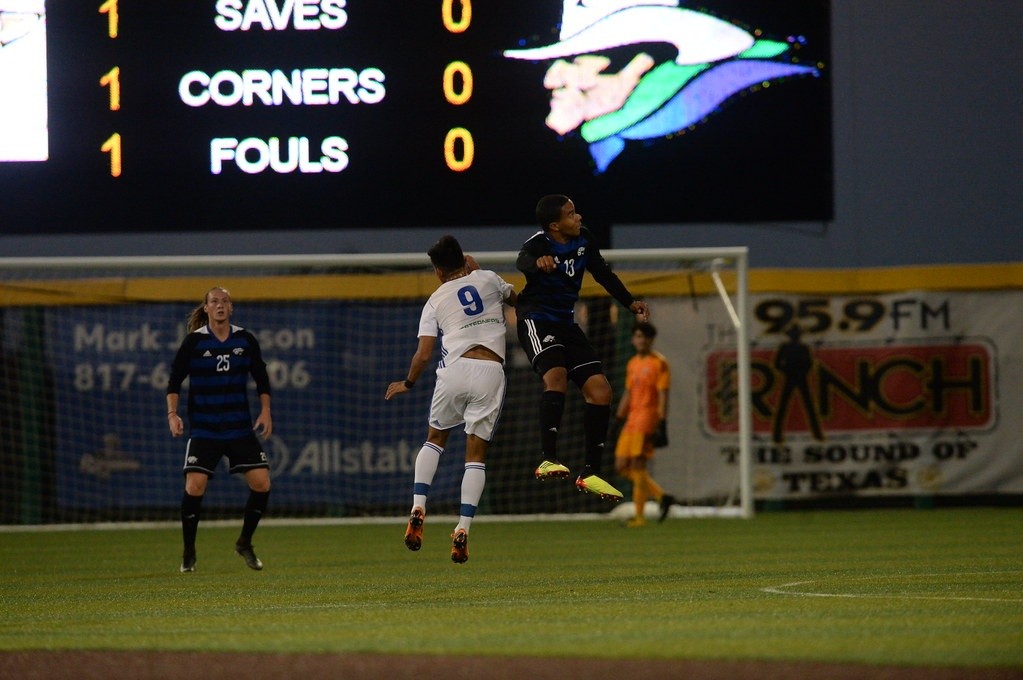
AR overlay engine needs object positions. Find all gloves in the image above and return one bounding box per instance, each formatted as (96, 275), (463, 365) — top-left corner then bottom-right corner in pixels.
(650, 420), (668, 448)
(608, 417), (626, 439)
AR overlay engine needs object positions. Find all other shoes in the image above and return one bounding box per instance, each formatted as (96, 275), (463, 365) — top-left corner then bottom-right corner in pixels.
(625, 518), (646, 528)
(657, 493), (674, 522)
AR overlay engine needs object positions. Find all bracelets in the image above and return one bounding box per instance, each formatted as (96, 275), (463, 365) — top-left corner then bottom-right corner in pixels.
(404, 379), (415, 389)
(168, 410), (177, 414)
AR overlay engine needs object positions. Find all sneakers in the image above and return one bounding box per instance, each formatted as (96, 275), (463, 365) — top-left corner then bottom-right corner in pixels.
(575, 474), (623, 501)
(180, 559), (195, 572)
(450, 528), (469, 565)
(403, 507), (425, 550)
(234, 545), (263, 570)
(536, 461), (570, 480)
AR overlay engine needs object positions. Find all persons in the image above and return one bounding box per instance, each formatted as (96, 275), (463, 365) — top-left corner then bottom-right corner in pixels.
(516, 193), (650, 502)
(385, 236), (517, 564)
(608, 319), (676, 528)
(166, 285), (273, 573)
